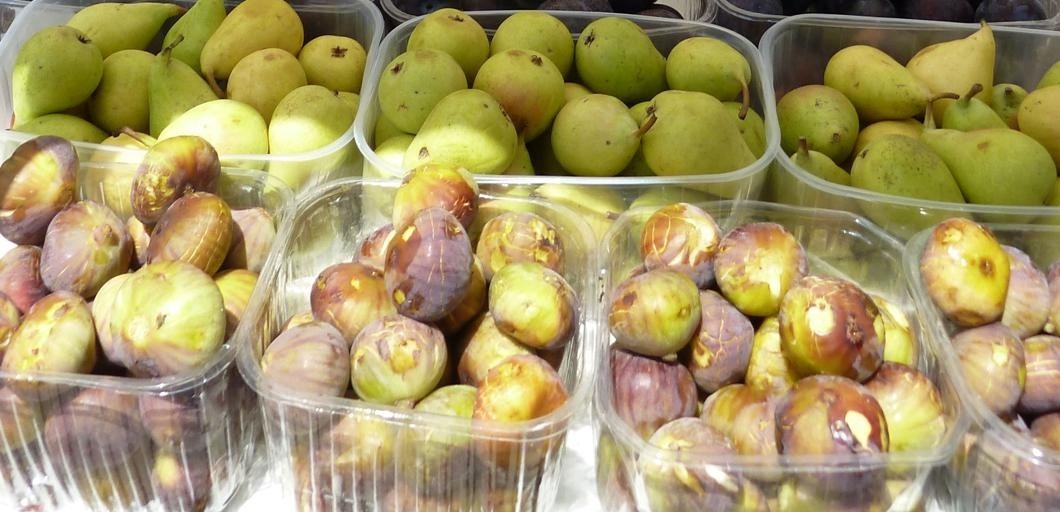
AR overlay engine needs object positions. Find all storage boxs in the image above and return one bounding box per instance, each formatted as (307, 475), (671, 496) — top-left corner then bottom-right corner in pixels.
(352, 7), (779, 216)
(0, 0), (384, 181)
(718, 0), (1058, 50)
(757, 12), (1058, 265)
(378, 0), (718, 47)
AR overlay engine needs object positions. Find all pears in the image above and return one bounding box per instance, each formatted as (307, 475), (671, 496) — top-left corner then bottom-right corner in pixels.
(7, 0), (369, 159)
(379, 9), (769, 207)
(776, 5), (1060, 208)
